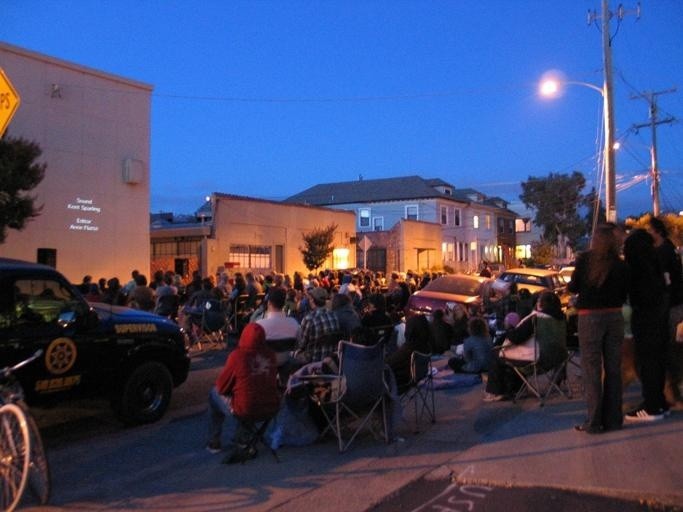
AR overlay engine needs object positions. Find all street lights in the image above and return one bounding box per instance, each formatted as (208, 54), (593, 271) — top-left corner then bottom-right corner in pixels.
(535, 71), (616, 222)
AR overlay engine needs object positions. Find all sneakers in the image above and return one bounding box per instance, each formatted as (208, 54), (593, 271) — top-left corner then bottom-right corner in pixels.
(228, 435), (248, 449)
(625, 405), (664, 423)
(204, 438), (223, 455)
(662, 406), (673, 417)
(573, 423), (589, 432)
(482, 392), (509, 403)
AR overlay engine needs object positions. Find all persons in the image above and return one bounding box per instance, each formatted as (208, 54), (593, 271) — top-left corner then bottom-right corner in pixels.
(567, 222), (631, 433)
(204, 322), (283, 453)
(448, 281), (567, 398)
(632, 218), (683, 412)
(81, 269), (452, 387)
(480, 262), (491, 279)
(619, 229), (672, 420)
(283, 326), (373, 448)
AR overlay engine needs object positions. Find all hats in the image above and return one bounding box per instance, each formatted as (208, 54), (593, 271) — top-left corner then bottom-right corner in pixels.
(307, 287), (329, 304)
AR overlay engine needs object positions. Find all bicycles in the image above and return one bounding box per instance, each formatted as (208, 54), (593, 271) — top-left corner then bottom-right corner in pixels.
(0, 402), (33, 511)
(0, 345), (51, 503)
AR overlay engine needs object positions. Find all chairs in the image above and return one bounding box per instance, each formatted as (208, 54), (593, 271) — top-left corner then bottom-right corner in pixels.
(233, 335), (435, 464)
(175, 294), (262, 352)
(491, 315), (579, 407)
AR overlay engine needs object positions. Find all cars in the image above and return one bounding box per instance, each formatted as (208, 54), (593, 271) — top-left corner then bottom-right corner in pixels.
(560, 266), (575, 284)
(493, 267), (571, 310)
(407, 273), (511, 319)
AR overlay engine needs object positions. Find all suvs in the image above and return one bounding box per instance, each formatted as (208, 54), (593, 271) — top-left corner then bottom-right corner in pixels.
(0, 256), (196, 429)
